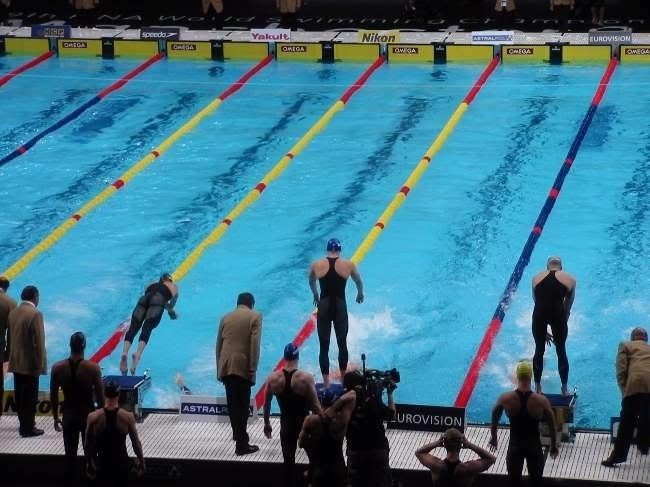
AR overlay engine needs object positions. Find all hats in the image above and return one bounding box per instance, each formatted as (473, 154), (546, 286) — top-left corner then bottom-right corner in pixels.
(326, 239), (343, 251)
(70, 332), (87, 350)
(104, 380), (120, 398)
(444, 428), (462, 446)
(283, 344), (299, 360)
(317, 387), (337, 408)
(548, 256), (561, 268)
(516, 363), (533, 380)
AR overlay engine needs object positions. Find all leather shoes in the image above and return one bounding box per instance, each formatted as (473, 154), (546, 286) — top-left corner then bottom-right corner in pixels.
(21, 429), (44, 437)
(236, 446), (259, 456)
(601, 457), (624, 466)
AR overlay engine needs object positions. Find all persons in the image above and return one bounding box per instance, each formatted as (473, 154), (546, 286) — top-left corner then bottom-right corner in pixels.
(264, 344), (326, 486)
(413, 427), (496, 486)
(83, 379), (146, 486)
(528, 255), (576, 398)
(491, 0), (519, 30)
(275, 0), (302, 29)
(201, 0), (226, 29)
(118, 272), (179, 374)
(174, 371), (191, 393)
(216, 292), (263, 454)
(340, 368), (396, 486)
(400, 0), (415, 17)
(308, 237), (364, 381)
(0, 277), (17, 412)
(294, 384), (357, 485)
(599, 326), (650, 466)
(6, 284), (47, 438)
(589, 0), (609, 25)
(550, 0), (576, 32)
(53, 332), (105, 486)
(71, 0), (104, 27)
(488, 362), (558, 486)
